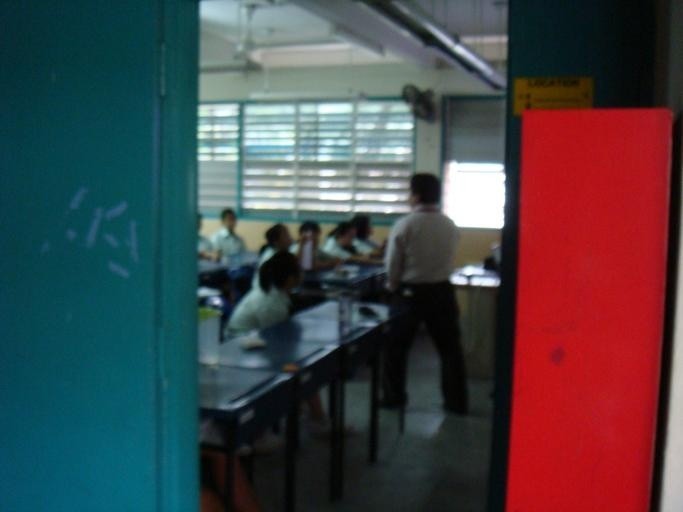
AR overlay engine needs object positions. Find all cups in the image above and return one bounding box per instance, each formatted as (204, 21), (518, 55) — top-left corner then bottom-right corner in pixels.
(336, 291), (354, 335)
(196, 307), (223, 366)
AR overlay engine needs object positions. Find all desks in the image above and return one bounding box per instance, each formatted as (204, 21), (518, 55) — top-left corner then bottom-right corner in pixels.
(195, 252), (500, 512)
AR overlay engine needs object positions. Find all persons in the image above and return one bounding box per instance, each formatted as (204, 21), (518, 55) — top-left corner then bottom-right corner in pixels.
(196, 210), (226, 307)
(349, 211), (388, 259)
(210, 206), (246, 308)
(199, 420), (261, 512)
(287, 218), (341, 273)
(378, 171), (470, 418)
(250, 218), (295, 288)
(220, 250), (363, 457)
(323, 220), (384, 268)
